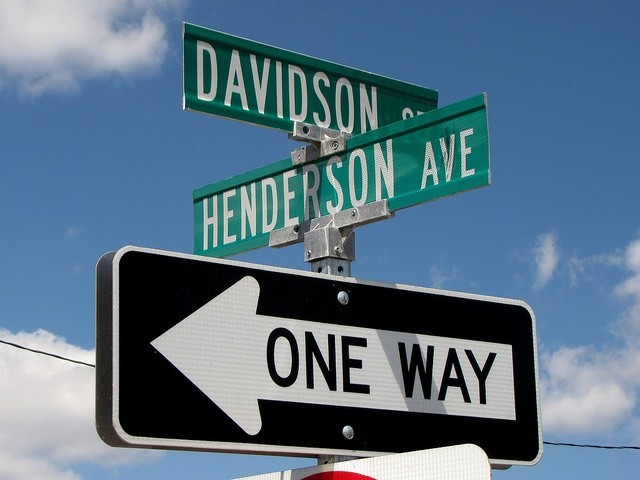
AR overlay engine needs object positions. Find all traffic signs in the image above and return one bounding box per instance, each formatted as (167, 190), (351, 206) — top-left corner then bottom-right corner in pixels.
(181, 21), (438, 146)
(192, 91), (491, 259)
(113, 245), (545, 467)
